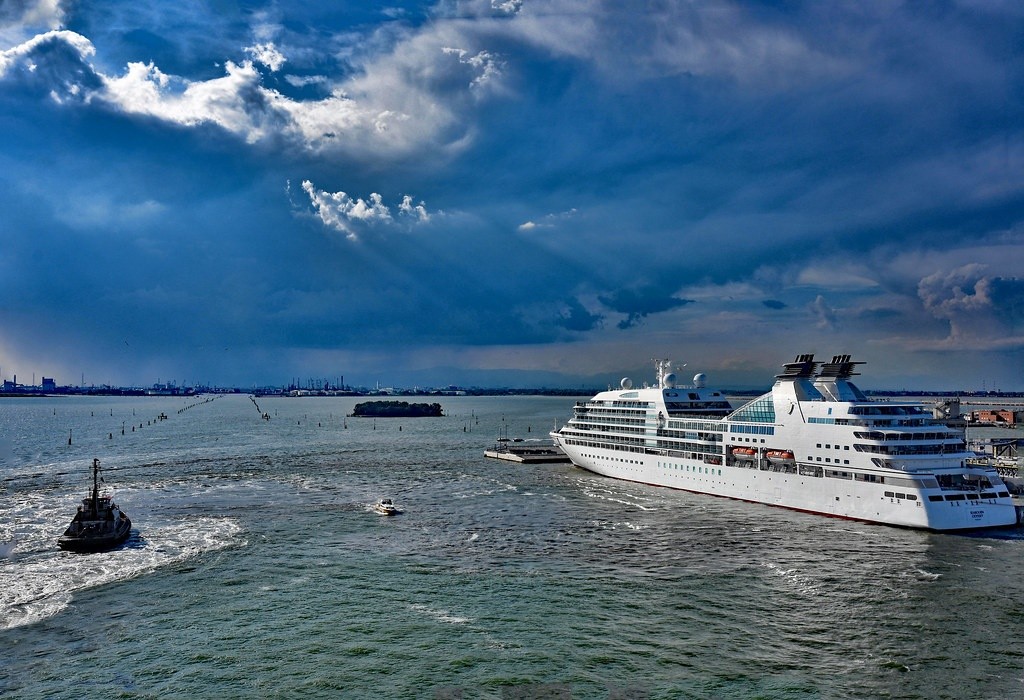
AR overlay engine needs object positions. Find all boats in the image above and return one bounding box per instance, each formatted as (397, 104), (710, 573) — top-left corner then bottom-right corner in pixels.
(925, 396), (1024, 499)
(376, 498), (397, 516)
(58, 458), (133, 553)
(548, 354), (1018, 534)
(347, 400), (444, 419)
